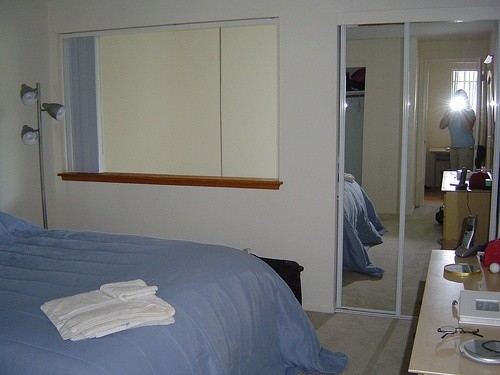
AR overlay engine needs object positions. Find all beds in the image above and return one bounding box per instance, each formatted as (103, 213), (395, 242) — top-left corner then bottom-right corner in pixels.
(343, 180), (388, 279)
(0, 211), (348, 375)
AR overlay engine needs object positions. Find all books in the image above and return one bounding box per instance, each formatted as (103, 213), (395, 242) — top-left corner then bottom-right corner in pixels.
(459, 290), (500, 326)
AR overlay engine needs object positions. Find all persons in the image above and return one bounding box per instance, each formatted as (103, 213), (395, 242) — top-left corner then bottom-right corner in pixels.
(439, 89), (477, 172)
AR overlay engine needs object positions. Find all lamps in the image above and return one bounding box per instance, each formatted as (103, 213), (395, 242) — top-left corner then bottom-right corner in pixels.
(20, 83), (66, 229)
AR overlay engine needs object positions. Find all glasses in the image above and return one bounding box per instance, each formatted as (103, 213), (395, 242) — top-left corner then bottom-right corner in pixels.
(438, 325), (484, 339)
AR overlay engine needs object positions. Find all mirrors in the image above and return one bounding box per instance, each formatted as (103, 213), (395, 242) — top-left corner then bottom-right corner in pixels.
(336, 18), (499, 316)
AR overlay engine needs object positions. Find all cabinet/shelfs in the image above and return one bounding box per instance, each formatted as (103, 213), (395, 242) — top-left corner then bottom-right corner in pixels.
(441, 171), (491, 250)
(408, 250), (500, 375)
